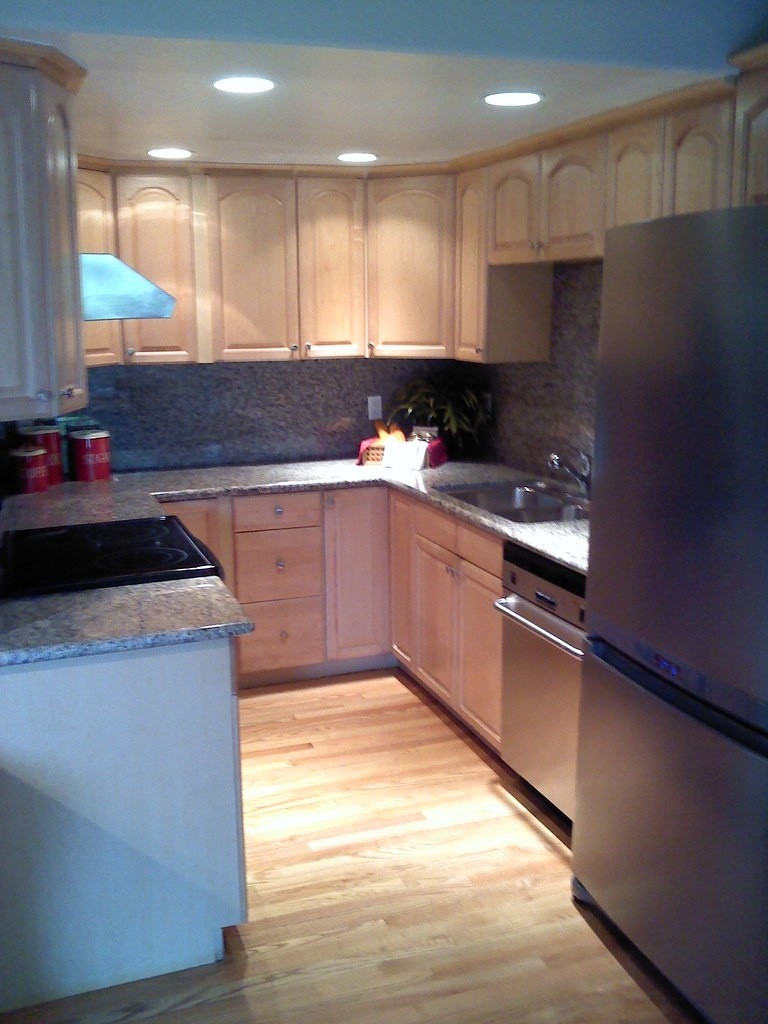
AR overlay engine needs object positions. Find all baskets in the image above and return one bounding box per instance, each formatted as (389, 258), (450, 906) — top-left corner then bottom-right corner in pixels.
(361, 444), (433, 468)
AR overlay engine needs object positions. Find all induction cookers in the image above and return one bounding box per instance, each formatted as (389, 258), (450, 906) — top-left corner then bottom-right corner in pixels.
(0, 514), (226, 604)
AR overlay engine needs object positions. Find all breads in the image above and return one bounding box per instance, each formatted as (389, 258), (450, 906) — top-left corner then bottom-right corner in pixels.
(373, 420), (406, 442)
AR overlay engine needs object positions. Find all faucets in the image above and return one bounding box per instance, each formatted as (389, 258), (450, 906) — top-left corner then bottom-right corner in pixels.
(547, 449), (593, 498)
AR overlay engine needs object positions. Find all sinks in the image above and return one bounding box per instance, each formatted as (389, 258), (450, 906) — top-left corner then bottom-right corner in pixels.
(430, 479), (590, 523)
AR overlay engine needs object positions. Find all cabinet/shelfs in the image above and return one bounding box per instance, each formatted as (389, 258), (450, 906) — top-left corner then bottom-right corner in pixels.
(158, 486), (504, 762)
(76, 42), (768, 367)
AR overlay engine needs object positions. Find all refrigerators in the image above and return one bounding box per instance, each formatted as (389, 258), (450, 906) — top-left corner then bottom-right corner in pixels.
(571, 213), (768, 1024)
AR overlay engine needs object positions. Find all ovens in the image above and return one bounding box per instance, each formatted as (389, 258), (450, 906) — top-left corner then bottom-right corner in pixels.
(497, 540), (587, 822)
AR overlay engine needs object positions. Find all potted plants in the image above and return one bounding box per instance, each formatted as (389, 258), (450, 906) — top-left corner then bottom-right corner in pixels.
(385, 366), (498, 448)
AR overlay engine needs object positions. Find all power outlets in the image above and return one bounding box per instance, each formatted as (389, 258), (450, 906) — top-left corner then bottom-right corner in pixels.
(367, 396), (383, 421)
